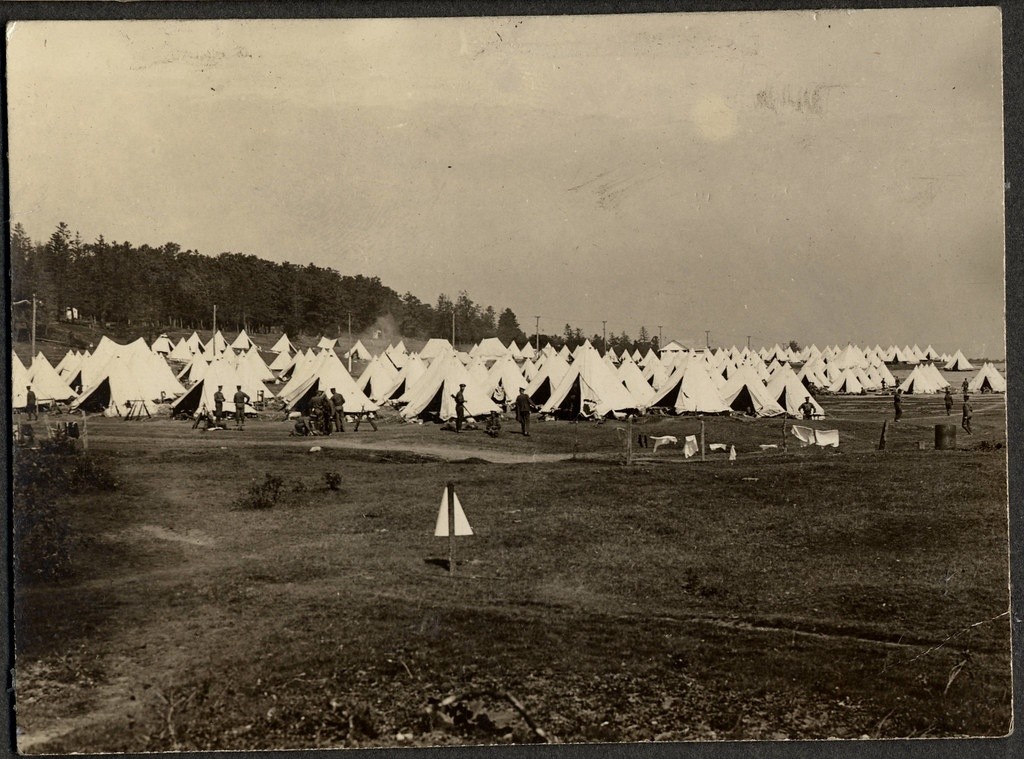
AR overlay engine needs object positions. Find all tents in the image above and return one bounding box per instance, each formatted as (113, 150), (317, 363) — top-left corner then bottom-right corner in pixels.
(12, 329), (1006, 419)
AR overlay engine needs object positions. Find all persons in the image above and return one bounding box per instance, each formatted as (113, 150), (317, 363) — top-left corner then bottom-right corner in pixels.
(962, 377), (969, 395)
(27, 385), (38, 421)
(881, 378), (885, 391)
(516, 387), (536, 436)
(798, 397), (816, 420)
(962, 395), (973, 434)
(895, 376), (900, 389)
(486, 411), (501, 438)
(944, 391), (953, 416)
(214, 385), (225, 423)
(894, 389), (904, 422)
(234, 386), (250, 426)
(861, 387), (867, 395)
(455, 384), (467, 433)
(289, 388), (346, 435)
